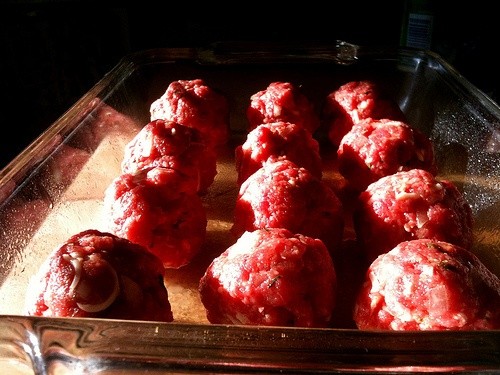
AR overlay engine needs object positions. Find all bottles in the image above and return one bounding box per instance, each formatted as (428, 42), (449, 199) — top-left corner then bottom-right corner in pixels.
(397, 0), (433, 72)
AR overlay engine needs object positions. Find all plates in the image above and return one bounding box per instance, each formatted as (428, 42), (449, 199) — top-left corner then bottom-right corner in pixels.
(0, 50), (499, 375)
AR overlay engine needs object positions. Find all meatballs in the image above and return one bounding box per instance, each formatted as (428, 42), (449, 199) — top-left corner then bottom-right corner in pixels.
(28, 79), (500, 332)
